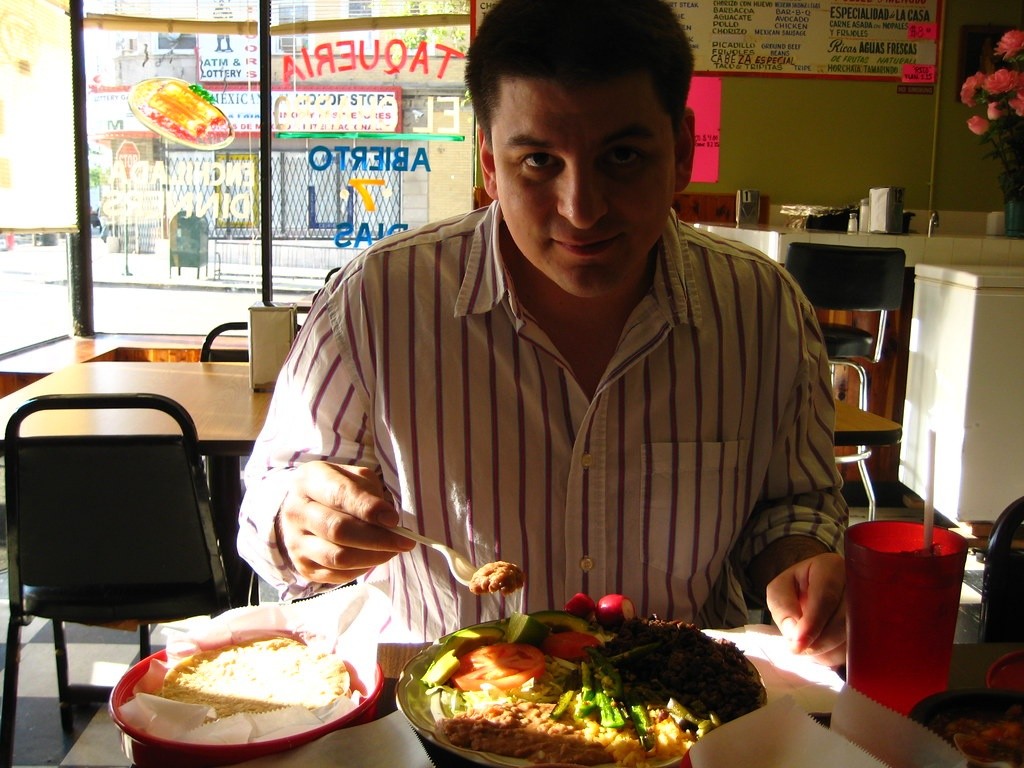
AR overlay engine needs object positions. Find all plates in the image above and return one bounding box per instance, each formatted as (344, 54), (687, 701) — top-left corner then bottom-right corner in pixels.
(108, 627), (385, 766)
(396, 601), (769, 768)
(909, 687), (1024, 767)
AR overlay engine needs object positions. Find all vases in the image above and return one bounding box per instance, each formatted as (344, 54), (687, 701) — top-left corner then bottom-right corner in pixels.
(1005, 202), (1024, 238)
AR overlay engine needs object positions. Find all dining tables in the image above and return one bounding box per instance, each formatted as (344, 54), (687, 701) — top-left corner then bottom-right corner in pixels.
(54, 624), (973, 768)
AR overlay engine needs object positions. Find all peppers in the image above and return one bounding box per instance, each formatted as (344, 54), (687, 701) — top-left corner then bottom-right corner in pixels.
(551, 640), (722, 757)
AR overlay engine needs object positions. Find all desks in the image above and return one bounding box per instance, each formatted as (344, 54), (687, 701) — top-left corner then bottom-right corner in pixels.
(1, 362), (290, 602)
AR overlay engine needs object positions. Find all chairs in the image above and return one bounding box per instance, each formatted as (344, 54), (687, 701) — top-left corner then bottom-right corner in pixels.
(0, 393), (235, 767)
(780, 240), (907, 406)
(201, 320), (301, 362)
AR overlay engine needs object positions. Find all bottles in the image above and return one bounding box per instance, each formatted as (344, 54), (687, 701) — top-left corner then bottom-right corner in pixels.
(859, 198), (869, 232)
(847, 213), (858, 232)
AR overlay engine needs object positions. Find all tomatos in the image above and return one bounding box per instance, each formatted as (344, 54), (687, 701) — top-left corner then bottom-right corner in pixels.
(451, 642), (545, 691)
(544, 631), (602, 659)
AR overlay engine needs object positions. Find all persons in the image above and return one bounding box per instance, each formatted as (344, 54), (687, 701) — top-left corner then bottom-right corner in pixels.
(237, 0), (851, 669)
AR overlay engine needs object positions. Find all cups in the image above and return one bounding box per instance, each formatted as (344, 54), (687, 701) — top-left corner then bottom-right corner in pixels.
(843, 519), (968, 726)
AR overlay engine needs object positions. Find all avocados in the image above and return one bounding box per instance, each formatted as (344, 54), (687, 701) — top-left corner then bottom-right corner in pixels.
(420, 610), (590, 688)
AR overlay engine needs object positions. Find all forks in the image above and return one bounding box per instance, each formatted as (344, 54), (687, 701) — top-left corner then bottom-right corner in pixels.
(390, 525), (478, 586)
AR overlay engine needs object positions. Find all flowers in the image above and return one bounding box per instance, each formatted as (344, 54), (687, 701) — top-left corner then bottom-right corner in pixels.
(960, 29), (1024, 201)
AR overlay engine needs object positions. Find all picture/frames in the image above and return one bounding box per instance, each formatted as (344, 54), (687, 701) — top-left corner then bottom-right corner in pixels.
(957, 24), (1016, 101)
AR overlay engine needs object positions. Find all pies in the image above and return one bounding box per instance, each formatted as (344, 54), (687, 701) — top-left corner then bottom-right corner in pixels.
(160, 634), (346, 723)
